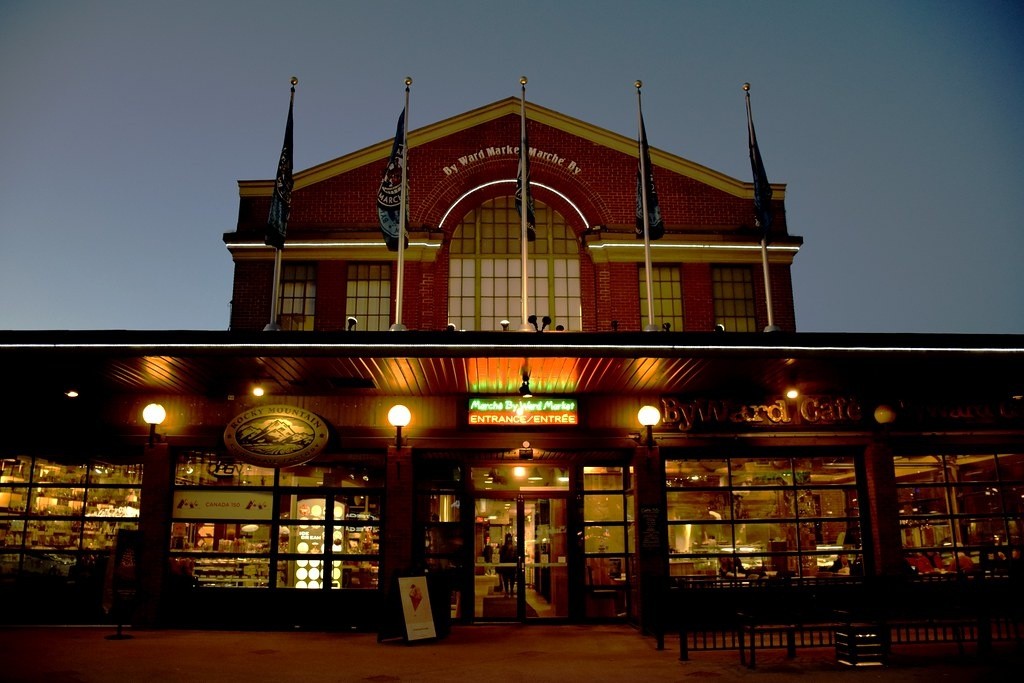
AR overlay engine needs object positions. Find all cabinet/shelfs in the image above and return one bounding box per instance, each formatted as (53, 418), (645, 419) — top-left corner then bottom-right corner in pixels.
(0, 456), (143, 583)
(174, 455), (379, 590)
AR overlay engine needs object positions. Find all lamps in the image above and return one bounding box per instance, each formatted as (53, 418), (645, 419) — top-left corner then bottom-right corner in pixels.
(638, 405), (661, 426)
(519, 385), (533, 399)
(874, 405), (897, 424)
(388, 404), (411, 427)
(141, 403), (167, 425)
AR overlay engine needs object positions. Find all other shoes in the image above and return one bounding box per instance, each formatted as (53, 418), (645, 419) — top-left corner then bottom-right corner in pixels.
(504, 594), (514, 600)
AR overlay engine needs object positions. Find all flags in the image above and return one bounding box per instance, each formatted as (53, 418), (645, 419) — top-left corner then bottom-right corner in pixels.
(515, 109), (538, 243)
(265, 85), (295, 251)
(634, 111), (665, 241)
(374, 104), (411, 252)
(745, 101), (777, 249)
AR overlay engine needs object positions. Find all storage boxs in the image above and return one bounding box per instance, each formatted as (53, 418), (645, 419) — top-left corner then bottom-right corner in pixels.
(701, 543), (765, 575)
(814, 544), (844, 567)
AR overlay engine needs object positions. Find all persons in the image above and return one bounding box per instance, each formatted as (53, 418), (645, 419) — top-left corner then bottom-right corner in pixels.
(827, 554), (853, 573)
(719, 556), (751, 579)
(496, 534), (518, 598)
